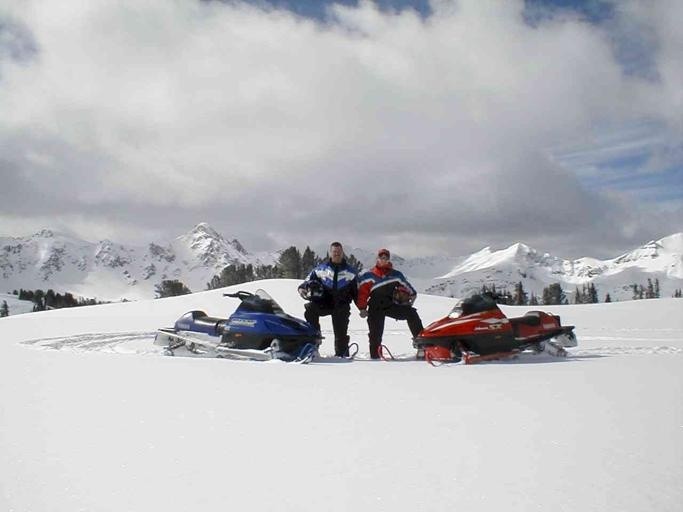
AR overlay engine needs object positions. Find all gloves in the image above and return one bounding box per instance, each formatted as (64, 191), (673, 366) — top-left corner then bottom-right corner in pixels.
(359, 309), (370, 318)
(409, 295), (417, 305)
(298, 287), (308, 300)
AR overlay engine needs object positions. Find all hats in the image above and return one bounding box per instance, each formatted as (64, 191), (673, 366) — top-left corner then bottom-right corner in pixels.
(376, 247), (390, 257)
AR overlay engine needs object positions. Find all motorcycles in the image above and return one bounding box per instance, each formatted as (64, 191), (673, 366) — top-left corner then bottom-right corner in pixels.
(415, 293), (579, 365)
(154, 287), (326, 366)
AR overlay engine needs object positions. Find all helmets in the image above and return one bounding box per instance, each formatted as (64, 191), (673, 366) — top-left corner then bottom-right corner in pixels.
(304, 280), (324, 299)
(392, 286), (411, 305)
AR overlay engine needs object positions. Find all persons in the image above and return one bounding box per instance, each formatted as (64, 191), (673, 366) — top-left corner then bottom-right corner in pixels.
(354, 247), (428, 359)
(297, 241), (357, 359)
